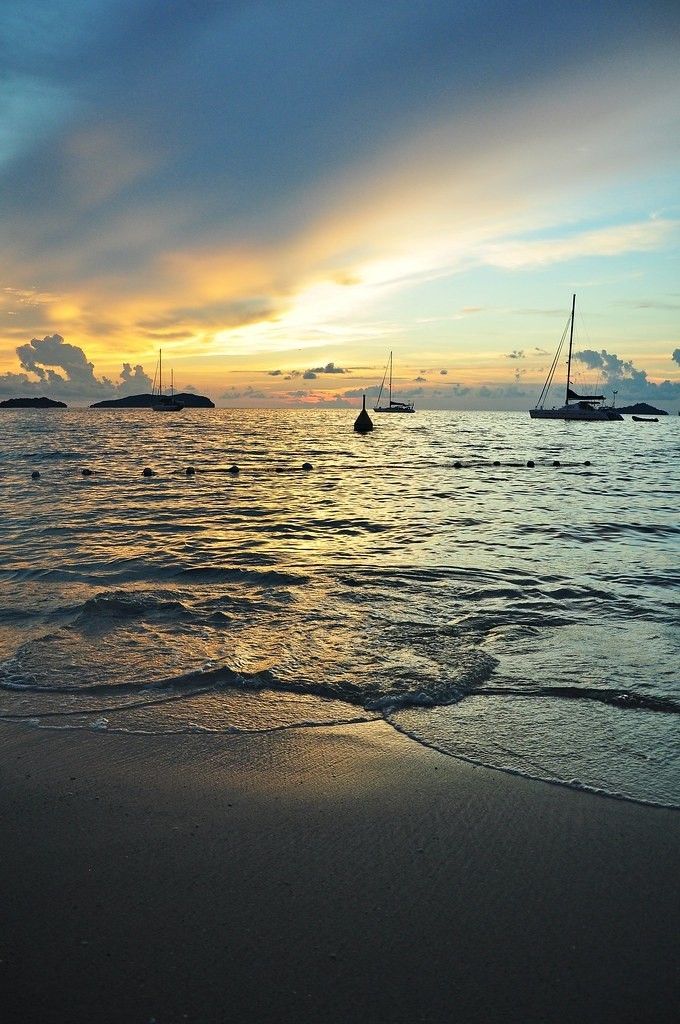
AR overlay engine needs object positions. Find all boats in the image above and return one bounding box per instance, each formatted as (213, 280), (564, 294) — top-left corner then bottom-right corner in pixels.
(353, 394), (374, 431)
(631, 415), (659, 422)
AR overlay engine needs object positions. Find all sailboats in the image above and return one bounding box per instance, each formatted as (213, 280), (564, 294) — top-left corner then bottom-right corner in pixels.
(372, 351), (416, 414)
(529, 294), (612, 421)
(150, 348), (185, 411)
